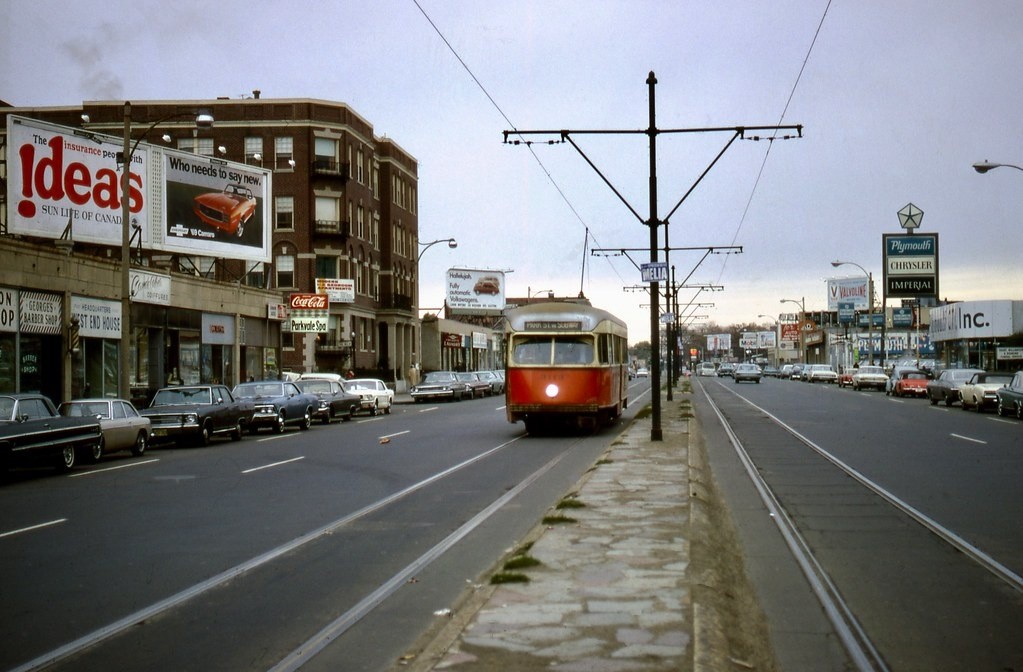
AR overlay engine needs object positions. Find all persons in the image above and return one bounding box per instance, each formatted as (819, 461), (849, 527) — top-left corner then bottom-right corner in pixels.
(408, 365), (417, 392)
(853, 362), (859, 368)
(453, 362), (465, 372)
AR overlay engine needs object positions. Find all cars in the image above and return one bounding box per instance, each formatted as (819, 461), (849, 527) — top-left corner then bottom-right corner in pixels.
(696, 362), (933, 398)
(344, 379), (395, 417)
(927, 369), (984, 408)
(474, 282), (500, 296)
(296, 380), (361, 425)
(139, 384), (255, 449)
(193, 183), (258, 238)
(411, 371), (466, 402)
(996, 370), (1023, 420)
(628, 367), (648, 381)
(478, 276), (499, 287)
(232, 382), (319, 436)
(457, 370), (505, 400)
(281, 372), (366, 390)
(958, 371), (1014, 413)
(58, 398), (152, 464)
(0, 394), (103, 484)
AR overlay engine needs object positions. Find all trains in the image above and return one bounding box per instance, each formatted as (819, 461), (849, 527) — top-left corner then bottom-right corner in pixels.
(502, 303), (627, 435)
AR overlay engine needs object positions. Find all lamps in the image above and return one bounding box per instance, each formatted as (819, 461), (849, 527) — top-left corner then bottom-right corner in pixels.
(68, 315), (81, 356)
(57, 114), (296, 166)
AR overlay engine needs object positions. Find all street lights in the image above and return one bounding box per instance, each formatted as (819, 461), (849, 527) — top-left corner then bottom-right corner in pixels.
(831, 262), (873, 366)
(121, 112), (213, 403)
(780, 300), (806, 364)
(758, 315), (779, 369)
